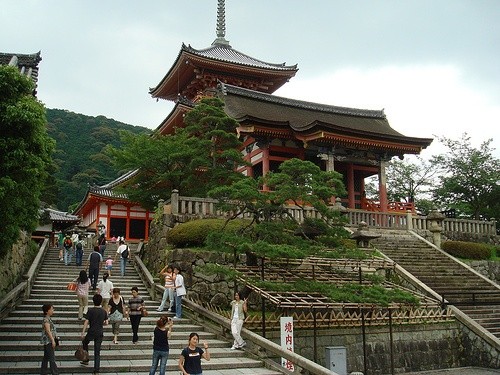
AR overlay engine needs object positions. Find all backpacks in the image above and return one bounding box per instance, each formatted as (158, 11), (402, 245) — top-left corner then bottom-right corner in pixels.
(76, 240), (82, 251)
(90, 253), (100, 265)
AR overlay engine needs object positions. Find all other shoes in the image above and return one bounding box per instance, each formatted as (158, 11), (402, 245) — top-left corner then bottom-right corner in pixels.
(80, 361), (89, 365)
(156, 309), (162, 312)
(167, 309), (171, 312)
(78, 317), (82, 320)
(231, 340), (247, 349)
(113, 339), (118, 344)
(174, 316), (181, 319)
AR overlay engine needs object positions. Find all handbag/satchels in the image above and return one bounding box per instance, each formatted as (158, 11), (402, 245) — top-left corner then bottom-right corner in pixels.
(121, 245), (129, 259)
(68, 280), (78, 291)
(141, 305), (147, 316)
(109, 310), (124, 324)
(74, 348), (89, 361)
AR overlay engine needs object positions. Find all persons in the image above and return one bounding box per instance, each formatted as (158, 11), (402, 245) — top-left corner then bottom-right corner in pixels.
(127, 287), (145, 343)
(40, 303), (58, 375)
(105, 255), (115, 276)
(87, 246), (103, 290)
(179, 333), (210, 375)
(54, 229), (83, 266)
(75, 270), (91, 320)
(115, 241), (131, 276)
(96, 233), (109, 257)
(107, 288), (126, 344)
(229, 291), (248, 350)
(149, 315), (173, 375)
(80, 294), (109, 375)
(96, 272), (114, 324)
(156, 264), (187, 319)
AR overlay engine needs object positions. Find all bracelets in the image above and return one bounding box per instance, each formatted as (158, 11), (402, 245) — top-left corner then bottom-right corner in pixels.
(205, 348), (208, 349)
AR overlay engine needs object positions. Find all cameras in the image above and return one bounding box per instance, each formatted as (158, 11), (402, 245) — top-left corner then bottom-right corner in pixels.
(168, 317), (172, 322)
